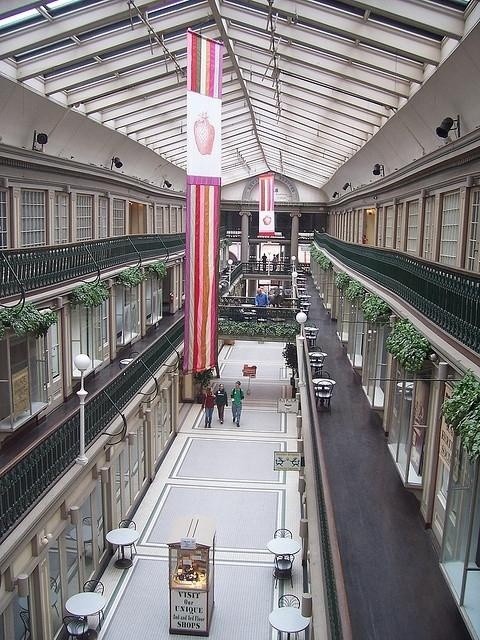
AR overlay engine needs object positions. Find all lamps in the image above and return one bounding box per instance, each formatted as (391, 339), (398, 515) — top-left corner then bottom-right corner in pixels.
(162, 180), (172, 188)
(331, 181), (352, 199)
(36, 132), (47, 152)
(435, 113), (461, 142)
(111, 157), (123, 170)
(372, 162), (385, 179)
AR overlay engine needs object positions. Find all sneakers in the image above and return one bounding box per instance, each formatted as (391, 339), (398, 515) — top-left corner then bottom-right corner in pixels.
(204, 417), (241, 428)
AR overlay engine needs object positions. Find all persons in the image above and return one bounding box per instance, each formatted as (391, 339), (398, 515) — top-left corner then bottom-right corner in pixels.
(260, 253), (268, 271)
(361, 234), (369, 244)
(231, 380), (245, 427)
(199, 387), (217, 428)
(269, 290), (285, 326)
(271, 254), (278, 272)
(254, 288), (267, 323)
(215, 383), (228, 425)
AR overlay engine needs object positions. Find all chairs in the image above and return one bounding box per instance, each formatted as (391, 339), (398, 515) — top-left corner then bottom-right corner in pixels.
(82, 516), (100, 553)
(20, 611), (31, 640)
(304, 323), (336, 413)
(264, 529), (311, 640)
(61, 580), (104, 640)
(118, 519), (136, 561)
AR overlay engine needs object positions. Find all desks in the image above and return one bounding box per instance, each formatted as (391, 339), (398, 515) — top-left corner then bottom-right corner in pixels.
(17, 589), (60, 619)
(296, 270), (312, 319)
(70, 525), (99, 561)
(105, 528), (139, 569)
